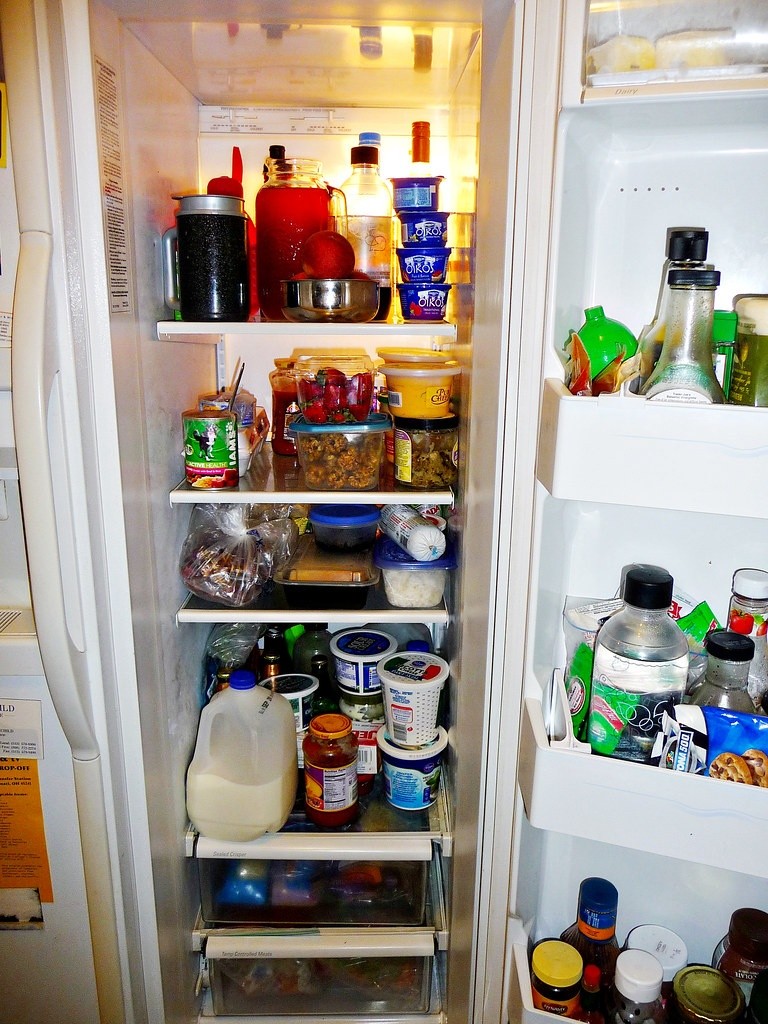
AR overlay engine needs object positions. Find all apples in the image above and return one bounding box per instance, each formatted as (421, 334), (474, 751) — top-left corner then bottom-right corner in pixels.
(288, 231), (373, 279)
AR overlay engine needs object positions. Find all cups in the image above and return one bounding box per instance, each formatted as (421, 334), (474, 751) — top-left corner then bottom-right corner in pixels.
(160, 194), (250, 322)
(375, 653), (449, 810)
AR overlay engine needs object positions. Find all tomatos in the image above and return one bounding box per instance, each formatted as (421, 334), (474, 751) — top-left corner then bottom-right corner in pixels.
(728, 609), (768, 636)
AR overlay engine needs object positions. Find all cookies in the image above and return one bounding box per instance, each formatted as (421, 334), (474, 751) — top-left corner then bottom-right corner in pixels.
(706, 749), (768, 789)
(298, 433), (460, 488)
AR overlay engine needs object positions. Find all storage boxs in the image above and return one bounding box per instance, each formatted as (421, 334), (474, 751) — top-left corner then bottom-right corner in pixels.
(272, 534), (380, 610)
(289, 411), (392, 490)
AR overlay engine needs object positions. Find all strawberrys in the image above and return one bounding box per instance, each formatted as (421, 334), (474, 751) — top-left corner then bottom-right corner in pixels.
(300, 367), (374, 423)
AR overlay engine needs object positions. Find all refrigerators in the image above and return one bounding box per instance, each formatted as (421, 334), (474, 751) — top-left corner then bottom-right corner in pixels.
(0, 1), (768, 1024)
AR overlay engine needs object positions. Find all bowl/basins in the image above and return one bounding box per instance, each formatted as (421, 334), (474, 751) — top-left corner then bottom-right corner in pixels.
(270, 505), (458, 607)
(388, 175), (453, 321)
(292, 345), (460, 490)
(258, 674), (320, 733)
(278, 279), (380, 323)
(329, 628), (398, 696)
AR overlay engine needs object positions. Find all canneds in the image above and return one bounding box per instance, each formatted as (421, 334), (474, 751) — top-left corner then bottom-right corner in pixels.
(301, 715), (358, 828)
(529, 923), (741, 1024)
(180, 410), (239, 490)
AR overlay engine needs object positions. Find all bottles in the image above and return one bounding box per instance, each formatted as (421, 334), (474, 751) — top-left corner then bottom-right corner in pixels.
(574, 231), (768, 406)
(400, 117), (442, 176)
(262, 142), (288, 183)
(528, 874), (767, 1024)
(269, 357), (297, 455)
(339, 117), (394, 321)
(186, 619), (361, 843)
(587, 564), (767, 767)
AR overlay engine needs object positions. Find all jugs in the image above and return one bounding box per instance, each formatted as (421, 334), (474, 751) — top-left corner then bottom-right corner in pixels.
(254, 157), (349, 321)
